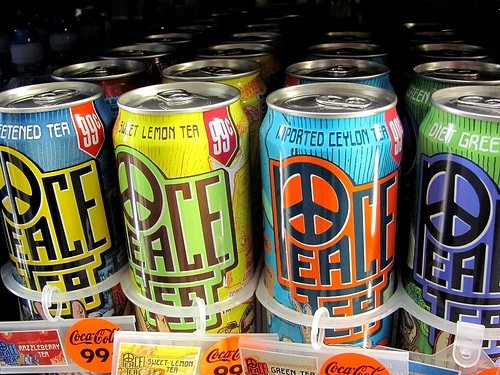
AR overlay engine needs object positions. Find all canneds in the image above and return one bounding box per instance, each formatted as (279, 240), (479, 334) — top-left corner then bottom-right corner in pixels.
(0, 8), (500, 367)
